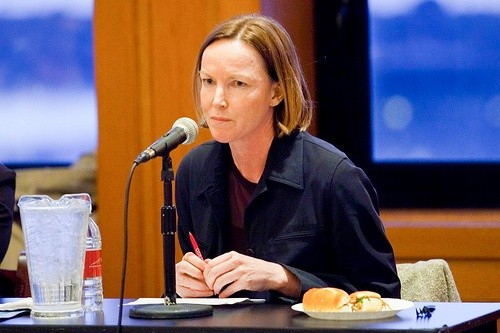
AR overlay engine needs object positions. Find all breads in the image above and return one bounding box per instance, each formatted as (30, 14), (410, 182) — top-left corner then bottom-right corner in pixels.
(302, 287), (351, 311)
(348, 291), (383, 313)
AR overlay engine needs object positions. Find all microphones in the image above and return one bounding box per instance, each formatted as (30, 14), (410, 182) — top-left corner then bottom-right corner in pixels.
(133, 116), (199, 166)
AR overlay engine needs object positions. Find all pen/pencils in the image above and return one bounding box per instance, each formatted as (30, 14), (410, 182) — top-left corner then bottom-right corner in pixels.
(189, 231), (217, 296)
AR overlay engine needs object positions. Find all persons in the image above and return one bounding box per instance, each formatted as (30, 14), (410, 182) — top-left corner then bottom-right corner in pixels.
(175, 14), (402, 306)
(0, 163), (17, 266)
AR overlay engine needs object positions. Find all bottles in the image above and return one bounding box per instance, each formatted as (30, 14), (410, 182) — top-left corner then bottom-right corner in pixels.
(80, 205), (103, 311)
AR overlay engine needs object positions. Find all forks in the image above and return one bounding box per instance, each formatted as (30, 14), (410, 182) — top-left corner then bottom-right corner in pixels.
(416, 305), (435, 317)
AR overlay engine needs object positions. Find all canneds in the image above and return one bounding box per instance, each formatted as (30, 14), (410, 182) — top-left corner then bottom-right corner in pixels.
(17, 252), (31, 298)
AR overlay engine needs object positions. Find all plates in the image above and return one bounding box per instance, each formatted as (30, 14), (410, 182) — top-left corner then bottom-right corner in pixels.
(291, 298), (416, 320)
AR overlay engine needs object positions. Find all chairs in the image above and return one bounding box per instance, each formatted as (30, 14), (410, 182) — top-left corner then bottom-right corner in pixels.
(396, 256), (462, 302)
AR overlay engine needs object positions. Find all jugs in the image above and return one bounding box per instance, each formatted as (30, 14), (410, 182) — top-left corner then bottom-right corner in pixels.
(15, 193), (92, 319)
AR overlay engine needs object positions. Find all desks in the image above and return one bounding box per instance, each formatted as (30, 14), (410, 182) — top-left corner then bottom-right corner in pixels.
(0, 293), (500, 333)
